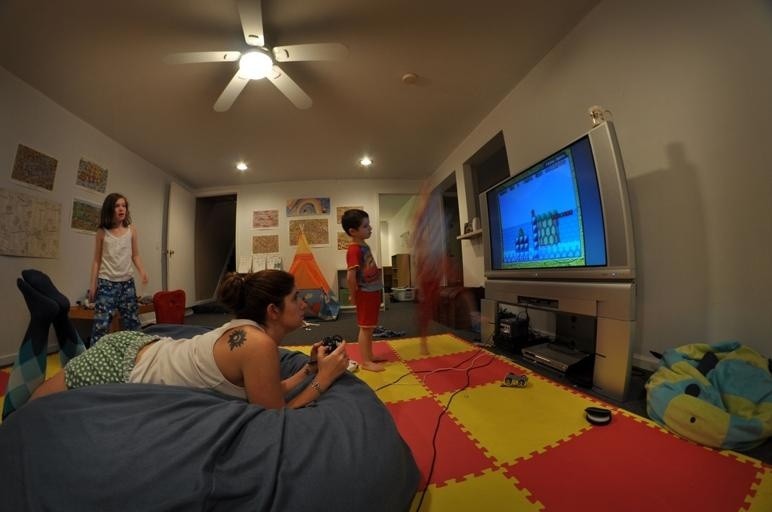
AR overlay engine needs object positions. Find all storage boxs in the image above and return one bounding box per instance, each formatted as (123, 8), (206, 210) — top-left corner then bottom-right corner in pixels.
(391, 287), (417, 302)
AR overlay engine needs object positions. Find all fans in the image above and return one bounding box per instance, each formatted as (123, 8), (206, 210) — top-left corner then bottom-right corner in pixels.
(162, 0), (349, 113)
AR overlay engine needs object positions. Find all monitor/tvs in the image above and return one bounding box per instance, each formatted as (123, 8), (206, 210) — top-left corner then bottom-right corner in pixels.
(479, 121), (636, 280)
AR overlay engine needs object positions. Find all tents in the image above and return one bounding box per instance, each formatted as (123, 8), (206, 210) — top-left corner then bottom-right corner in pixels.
(290, 224), (342, 322)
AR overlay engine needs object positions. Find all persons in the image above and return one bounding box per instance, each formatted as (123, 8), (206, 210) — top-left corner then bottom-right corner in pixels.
(88, 192), (149, 347)
(341, 209), (387, 372)
(2, 268), (350, 426)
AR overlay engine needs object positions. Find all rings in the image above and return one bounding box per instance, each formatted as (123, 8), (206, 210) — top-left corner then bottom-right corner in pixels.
(346, 363), (348, 366)
(341, 352), (345, 356)
(343, 361), (346, 364)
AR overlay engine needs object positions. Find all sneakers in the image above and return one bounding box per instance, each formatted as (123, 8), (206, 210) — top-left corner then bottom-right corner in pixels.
(373, 325), (407, 338)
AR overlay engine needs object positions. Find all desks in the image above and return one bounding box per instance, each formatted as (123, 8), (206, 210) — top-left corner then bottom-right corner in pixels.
(68, 304), (155, 334)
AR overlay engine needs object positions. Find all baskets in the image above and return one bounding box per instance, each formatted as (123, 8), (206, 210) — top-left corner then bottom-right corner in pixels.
(390, 286), (415, 301)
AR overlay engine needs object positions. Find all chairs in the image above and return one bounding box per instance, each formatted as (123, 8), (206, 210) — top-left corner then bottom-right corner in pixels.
(142, 290), (185, 329)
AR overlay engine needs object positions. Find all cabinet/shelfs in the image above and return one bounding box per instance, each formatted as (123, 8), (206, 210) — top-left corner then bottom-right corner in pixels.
(480, 279), (638, 404)
(383, 254), (411, 288)
(335, 268), (386, 313)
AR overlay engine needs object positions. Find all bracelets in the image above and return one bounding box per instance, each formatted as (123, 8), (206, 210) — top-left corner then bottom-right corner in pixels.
(310, 380), (323, 394)
